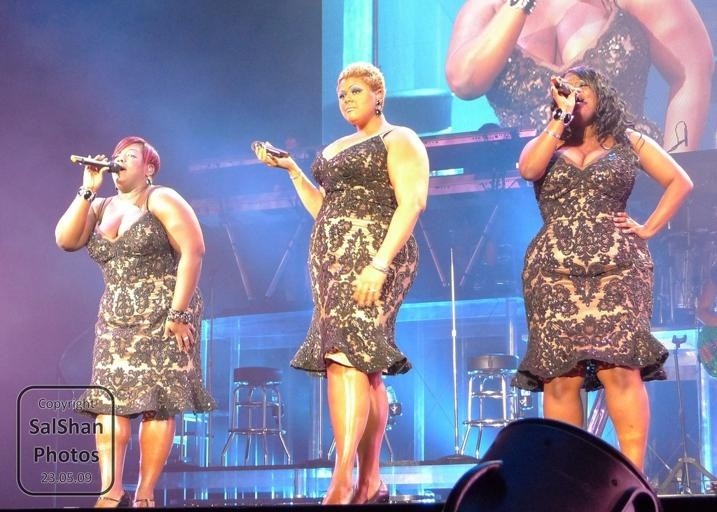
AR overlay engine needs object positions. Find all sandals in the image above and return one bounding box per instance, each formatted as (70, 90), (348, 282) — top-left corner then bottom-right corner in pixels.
(97, 490), (131, 508)
(133, 499), (155, 508)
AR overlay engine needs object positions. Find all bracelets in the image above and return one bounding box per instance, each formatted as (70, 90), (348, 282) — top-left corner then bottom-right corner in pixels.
(370, 259), (390, 277)
(543, 127), (562, 141)
(509, 0), (537, 14)
(287, 170), (303, 181)
(77, 182), (97, 202)
(165, 307), (194, 327)
(551, 106), (577, 126)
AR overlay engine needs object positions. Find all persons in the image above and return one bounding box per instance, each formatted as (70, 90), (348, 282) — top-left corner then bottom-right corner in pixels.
(514, 63), (696, 481)
(54, 133), (219, 511)
(443, 0), (716, 154)
(254, 60), (432, 510)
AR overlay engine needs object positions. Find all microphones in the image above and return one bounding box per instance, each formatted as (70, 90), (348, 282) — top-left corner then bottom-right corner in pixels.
(684, 127), (688, 147)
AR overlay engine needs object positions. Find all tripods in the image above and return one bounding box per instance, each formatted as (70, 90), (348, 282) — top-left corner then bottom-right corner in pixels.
(550, 76), (579, 102)
(251, 140), (289, 158)
(70, 154), (121, 173)
(657, 349), (717, 495)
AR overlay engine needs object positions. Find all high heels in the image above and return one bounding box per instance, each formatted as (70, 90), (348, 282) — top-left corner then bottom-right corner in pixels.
(365, 480), (390, 505)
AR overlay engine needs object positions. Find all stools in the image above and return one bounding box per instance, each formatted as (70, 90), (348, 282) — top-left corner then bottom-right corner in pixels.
(221, 366), (292, 466)
(166, 410), (213, 468)
(326, 431), (393, 463)
(461, 354), (522, 460)
